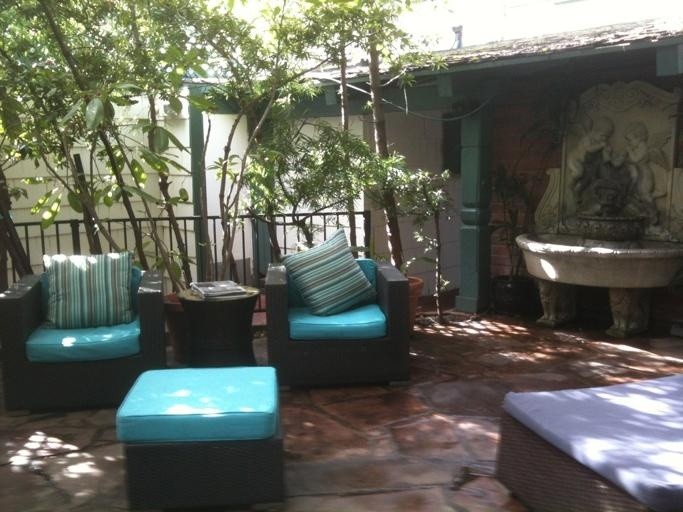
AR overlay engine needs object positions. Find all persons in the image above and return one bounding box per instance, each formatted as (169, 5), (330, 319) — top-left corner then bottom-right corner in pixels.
(623, 121), (659, 225)
(565, 115), (614, 188)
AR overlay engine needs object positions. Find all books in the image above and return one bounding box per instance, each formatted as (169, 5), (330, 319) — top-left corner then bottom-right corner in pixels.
(191, 280), (247, 300)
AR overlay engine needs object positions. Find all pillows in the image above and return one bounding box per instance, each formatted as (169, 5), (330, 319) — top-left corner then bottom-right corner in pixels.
(279, 226), (375, 314)
(42, 251), (134, 326)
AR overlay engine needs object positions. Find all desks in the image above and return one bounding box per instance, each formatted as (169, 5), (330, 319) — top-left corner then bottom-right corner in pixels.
(164, 284), (259, 366)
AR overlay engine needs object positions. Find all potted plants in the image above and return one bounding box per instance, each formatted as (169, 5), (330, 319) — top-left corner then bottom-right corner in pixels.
(490, 170), (540, 316)
(395, 252), (437, 331)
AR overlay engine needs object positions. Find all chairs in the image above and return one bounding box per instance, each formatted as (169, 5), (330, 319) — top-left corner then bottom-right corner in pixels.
(260, 259), (408, 390)
(0, 257), (165, 415)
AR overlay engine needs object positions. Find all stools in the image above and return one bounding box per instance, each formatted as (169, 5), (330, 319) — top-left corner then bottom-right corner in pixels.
(115, 364), (284, 510)
(493, 373), (683, 511)
